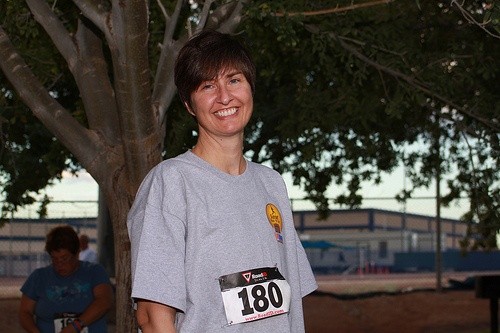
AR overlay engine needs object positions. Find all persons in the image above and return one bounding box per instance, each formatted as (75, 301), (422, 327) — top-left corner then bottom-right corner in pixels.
(20, 225), (117, 333)
(78, 234), (100, 264)
(126, 28), (318, 333)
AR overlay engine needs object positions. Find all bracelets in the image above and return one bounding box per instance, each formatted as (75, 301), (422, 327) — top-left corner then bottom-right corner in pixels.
(63, 318), (85, 333)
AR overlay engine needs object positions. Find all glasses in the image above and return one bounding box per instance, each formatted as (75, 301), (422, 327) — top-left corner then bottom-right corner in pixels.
(48, 254), (74, 266)
